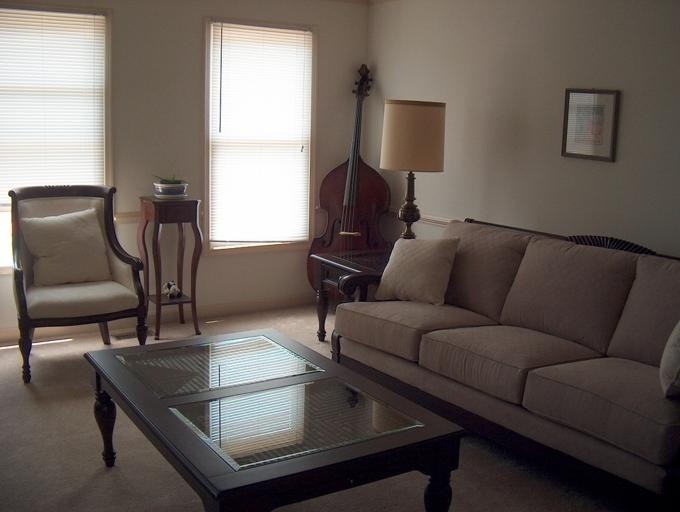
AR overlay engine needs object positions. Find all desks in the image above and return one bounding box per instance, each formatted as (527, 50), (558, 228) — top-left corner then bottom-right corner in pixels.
(135, 196), (203, 340)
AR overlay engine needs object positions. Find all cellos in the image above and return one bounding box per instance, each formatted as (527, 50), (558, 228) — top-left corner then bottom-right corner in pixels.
(307, 64), (392, 301)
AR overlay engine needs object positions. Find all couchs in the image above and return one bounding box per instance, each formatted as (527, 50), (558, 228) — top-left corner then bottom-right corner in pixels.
(331, 219), (679, 512)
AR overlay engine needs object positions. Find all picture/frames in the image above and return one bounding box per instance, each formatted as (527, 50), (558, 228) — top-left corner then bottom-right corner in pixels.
(559, 88), (621, 162)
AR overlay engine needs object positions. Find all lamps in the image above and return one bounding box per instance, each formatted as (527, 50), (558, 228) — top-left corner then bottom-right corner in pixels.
(379, 99), (444, 239)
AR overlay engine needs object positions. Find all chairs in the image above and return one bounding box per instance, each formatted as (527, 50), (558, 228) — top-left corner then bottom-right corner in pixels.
(7, 184), (150, 383)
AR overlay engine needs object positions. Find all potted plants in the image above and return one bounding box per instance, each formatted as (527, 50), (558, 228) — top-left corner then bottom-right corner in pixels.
(152, 174), (190, 201)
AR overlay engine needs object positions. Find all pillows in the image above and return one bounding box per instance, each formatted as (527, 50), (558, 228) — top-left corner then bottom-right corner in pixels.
(375, 237), (460, 307)
(658, 320), (679, 399)
(18, 207), (111, 287)
